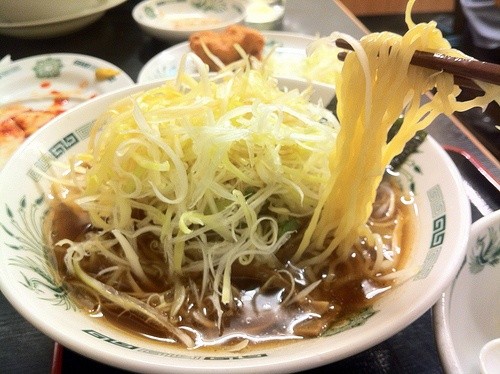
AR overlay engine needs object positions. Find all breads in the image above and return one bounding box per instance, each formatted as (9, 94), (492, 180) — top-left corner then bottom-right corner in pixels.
(189, 24), (265, 71)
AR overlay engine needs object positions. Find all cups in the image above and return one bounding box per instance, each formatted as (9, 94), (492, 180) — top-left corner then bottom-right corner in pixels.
(244, 0), (287, 30)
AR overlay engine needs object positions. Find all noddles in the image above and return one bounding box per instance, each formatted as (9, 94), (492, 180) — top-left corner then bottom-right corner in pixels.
(31, 0), (500, 353)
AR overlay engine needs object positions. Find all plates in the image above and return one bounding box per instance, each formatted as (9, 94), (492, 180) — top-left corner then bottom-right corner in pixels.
(136, 32), (352, 85)
(0, 53), (137, 173)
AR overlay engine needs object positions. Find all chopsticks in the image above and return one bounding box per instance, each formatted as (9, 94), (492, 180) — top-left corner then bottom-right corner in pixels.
(336, 38), (500, 86)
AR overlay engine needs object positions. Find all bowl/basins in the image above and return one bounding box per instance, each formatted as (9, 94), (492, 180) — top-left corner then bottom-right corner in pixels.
(0, 74), (471, 374)
(433, 209), (500, 374)
(134, 0), (247, 43)
(0, 0), (128, 39)
